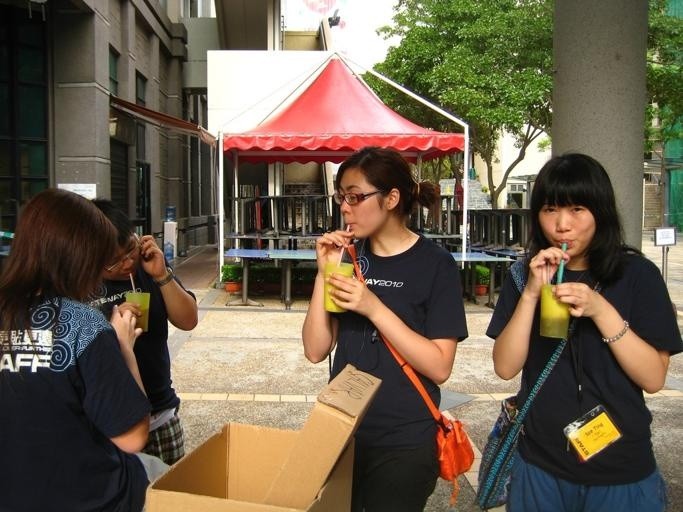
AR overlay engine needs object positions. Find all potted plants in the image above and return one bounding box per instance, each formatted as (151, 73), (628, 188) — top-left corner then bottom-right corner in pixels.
(471, 264), (490, 296)
(458, 269), (471, 297)
(221, 264), (317, 295)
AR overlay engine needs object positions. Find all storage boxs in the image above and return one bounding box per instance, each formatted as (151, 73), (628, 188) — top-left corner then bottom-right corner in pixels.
(145, 363), (384, 512)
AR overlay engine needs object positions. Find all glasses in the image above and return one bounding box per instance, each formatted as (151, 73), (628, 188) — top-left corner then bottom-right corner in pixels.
(333, 191), (379, 206)
(103, 232), (141, 272)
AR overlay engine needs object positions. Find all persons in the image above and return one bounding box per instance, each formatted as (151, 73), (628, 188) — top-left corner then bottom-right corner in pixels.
(302, 145), (468, 512)
(86, 197), (198, 466)
(486, 152), (683, 511)
(1, 187), (152, 512)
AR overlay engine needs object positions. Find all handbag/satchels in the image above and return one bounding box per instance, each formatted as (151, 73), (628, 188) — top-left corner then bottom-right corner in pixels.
(435, 418), (474, 481)
(475, 395), (524, 510)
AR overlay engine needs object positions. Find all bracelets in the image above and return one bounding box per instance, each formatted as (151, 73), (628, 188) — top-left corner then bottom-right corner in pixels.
(152, 267), (173, 287)
(602, 319), (629, 343)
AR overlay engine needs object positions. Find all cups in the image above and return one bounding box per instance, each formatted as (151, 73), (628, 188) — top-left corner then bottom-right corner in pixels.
(323, 262), (355, 314)
(540, 285), (570, 339)
(125, 293), (150, 333)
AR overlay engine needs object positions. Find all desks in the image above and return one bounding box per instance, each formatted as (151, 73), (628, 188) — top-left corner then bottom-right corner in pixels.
(269, 249), (318, 310)
(450, 252), (517, 310)
(223, 249), (269, 308)
